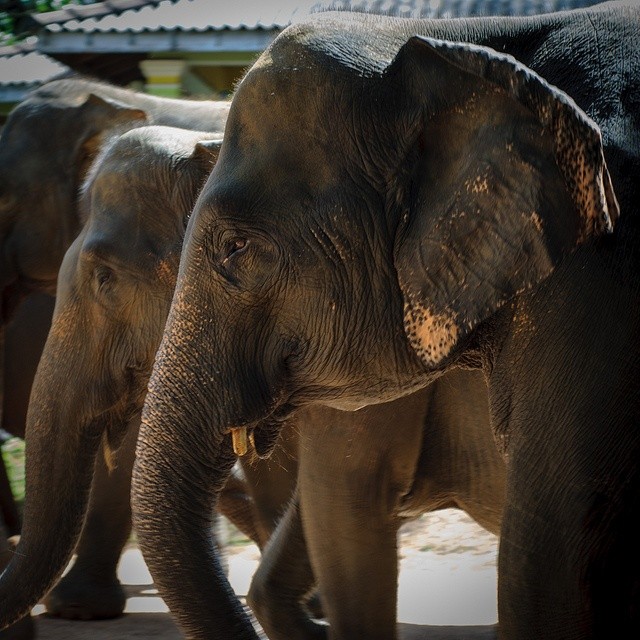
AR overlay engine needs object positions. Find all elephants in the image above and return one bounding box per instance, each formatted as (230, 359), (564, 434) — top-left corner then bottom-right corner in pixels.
(1, 73), (261, 623)
(0, 123), (504, 640)
(129, 0), (640, 640)
